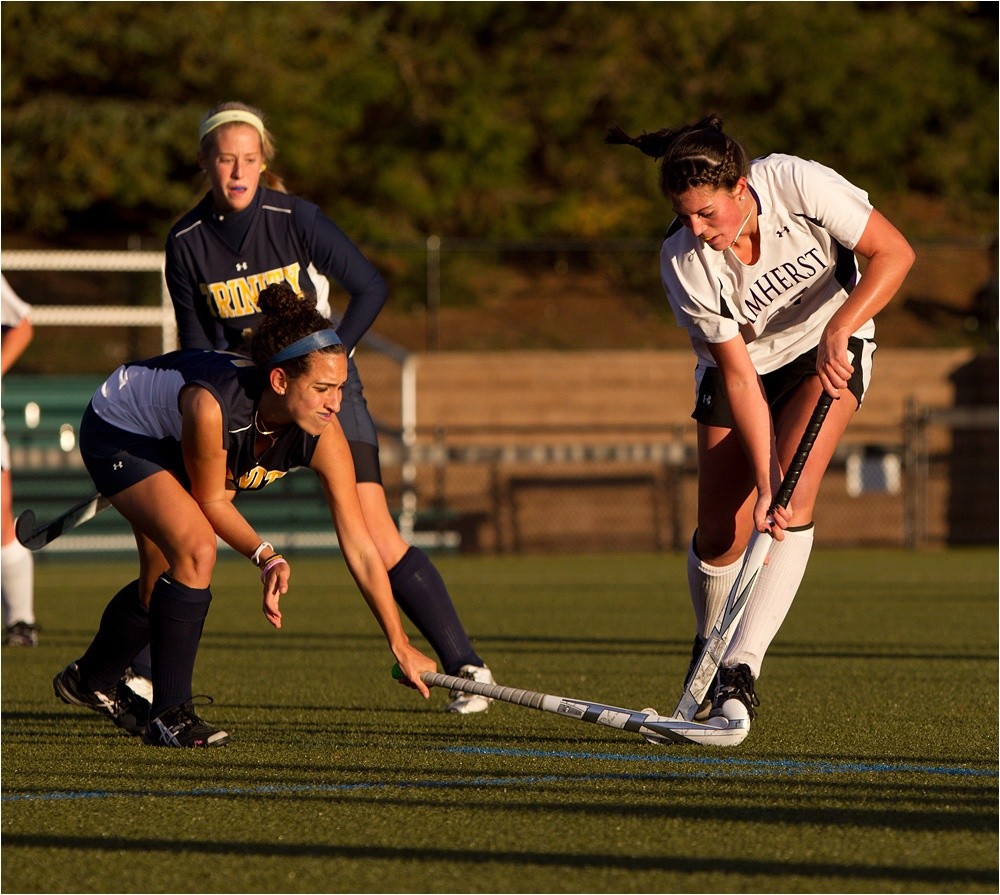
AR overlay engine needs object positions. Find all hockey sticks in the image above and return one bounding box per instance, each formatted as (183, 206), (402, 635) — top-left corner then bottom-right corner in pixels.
(391, 663), (751, 746)
(640, 349), (855, 745)
(15, 492), (113, 551)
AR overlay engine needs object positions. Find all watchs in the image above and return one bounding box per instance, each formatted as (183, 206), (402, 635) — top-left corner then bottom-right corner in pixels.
(250, 541), (274, 566)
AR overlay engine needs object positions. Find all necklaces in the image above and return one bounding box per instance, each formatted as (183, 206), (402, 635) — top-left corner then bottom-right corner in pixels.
(732, 205), (753, 252)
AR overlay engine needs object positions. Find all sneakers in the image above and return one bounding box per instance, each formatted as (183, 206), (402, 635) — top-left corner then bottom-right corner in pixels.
(53, 657), (150, 735)
(683, 634), (761, 732)
(143, 694), (231, 748)
(446, 665), (498, 714)
(3, 621), (40, 649)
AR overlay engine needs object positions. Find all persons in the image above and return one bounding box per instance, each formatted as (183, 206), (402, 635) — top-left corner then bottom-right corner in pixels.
(1, 278), (42, 648)
(603, 114), (917, 731)
(51, 280), (437, 747)
(124, 101), (498, 715)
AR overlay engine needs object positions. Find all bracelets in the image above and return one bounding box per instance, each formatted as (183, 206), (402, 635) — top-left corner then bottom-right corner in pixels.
(259, 554), (287, 583)
